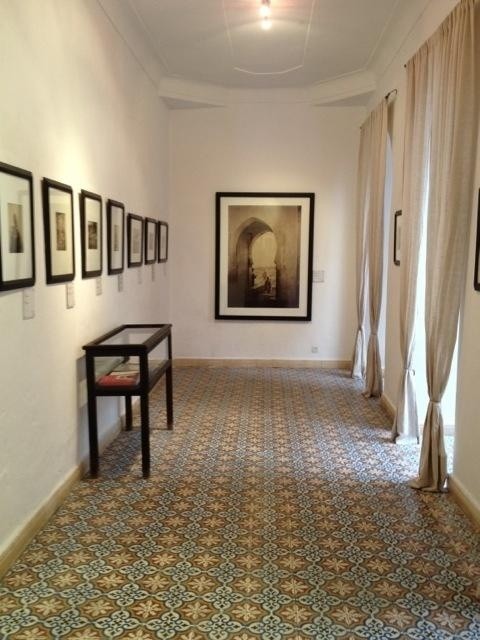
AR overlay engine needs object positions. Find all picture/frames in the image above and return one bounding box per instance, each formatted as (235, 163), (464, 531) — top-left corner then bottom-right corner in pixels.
(0, 161), (169, 292)
(214, 191), (316, 321)
